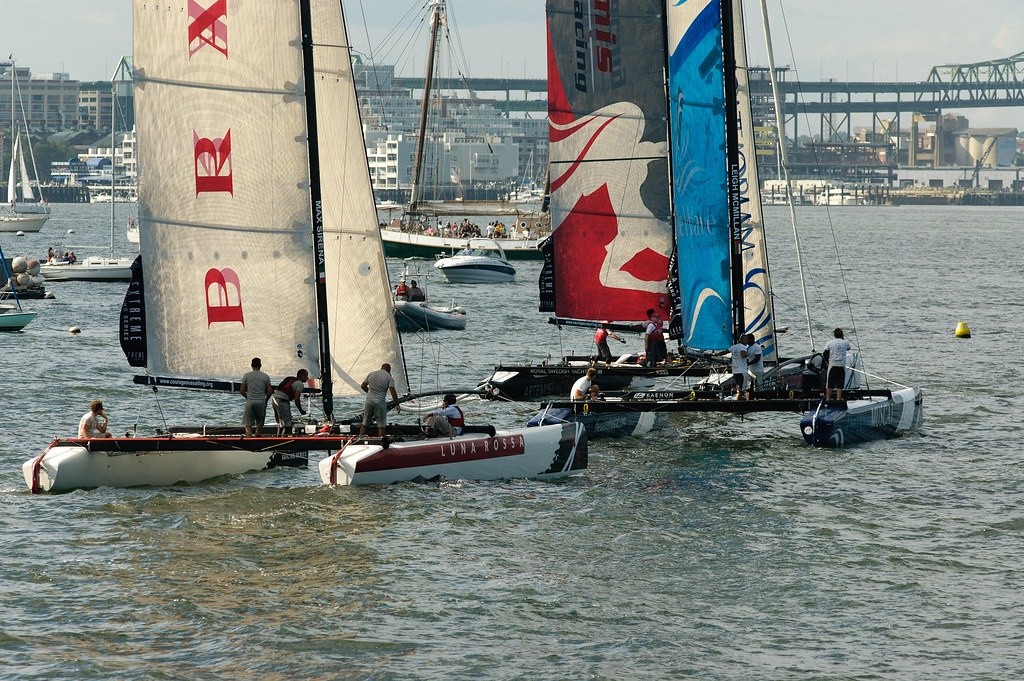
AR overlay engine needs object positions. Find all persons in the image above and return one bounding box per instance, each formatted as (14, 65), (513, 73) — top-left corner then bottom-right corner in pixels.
(272, 369), (309, 434)
(78, 401), (111, 438)
(592, 320), (626, 367)
(823, 327), (851, 401)
(634, 309), (668, 363)
(48, 247), (53, 262)
(415, 395), (464, 440)
(240, 358), (272, 434)
(57, 251), (76, 264)
(418, 221), (424, 231)
(463, 244), (472, 256)
(381, 220), (387, 226)
(361, 363), (401, 437)
(713, 334), (764, 401)
(391, 217), (395, 224)
(437, 218), (481, 238)
(397, 280), (422, 301)
(486, 220), (507, 238)
(428, 224), (434, 235)
(571, 368), (606, 403)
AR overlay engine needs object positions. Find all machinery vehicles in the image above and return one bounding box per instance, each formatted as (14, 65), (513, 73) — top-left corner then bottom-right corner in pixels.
(968, 136), (998, 183)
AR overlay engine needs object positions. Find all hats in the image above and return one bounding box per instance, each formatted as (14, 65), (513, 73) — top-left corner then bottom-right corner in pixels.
(400, 278), (405, 283)
(411, 280), (415, 284)
(651, 313), (661, 318)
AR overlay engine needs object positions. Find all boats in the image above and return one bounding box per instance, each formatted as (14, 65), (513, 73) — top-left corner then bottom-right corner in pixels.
(762, 186), (861, 207)
(432, 237), (518, 286)
(391, 262), (467, 332)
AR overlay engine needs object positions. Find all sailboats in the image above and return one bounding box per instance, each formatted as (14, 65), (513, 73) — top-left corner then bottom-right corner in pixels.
(36, 81), (140, 281)
(0, 245), (38, 332)
(365, 1), (555, 261)
(18, 1), (590, 499)
(0, 53), (52, 233)
(474, 1), (923, 447)
(504, 148), (547, 203)
